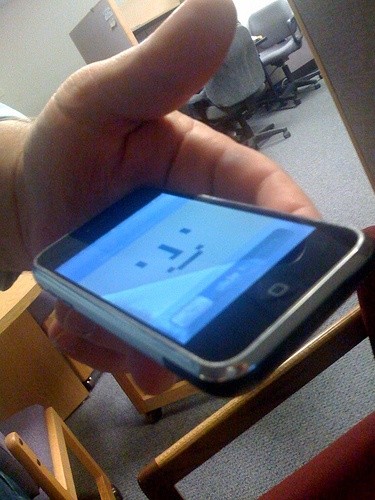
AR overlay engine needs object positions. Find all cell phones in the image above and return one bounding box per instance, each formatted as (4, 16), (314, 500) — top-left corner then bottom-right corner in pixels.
(31, 181), (374, 400)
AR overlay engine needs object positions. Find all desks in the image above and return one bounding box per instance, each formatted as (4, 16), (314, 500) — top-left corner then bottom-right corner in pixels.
(251, 36), (288, 109)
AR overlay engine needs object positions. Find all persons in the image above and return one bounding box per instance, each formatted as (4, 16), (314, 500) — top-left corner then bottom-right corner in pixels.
(0, 0), (325, 394)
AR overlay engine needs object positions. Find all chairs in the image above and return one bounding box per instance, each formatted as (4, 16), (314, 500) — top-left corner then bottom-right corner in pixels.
(138, 226), (375, 500)
(0, 403), (125, 500)
(248, 1), (323, 109)
(184, 23), (291, 151)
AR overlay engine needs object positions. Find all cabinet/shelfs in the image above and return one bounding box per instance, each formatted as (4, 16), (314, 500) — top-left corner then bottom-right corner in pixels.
(0, 271), (94, 421)
